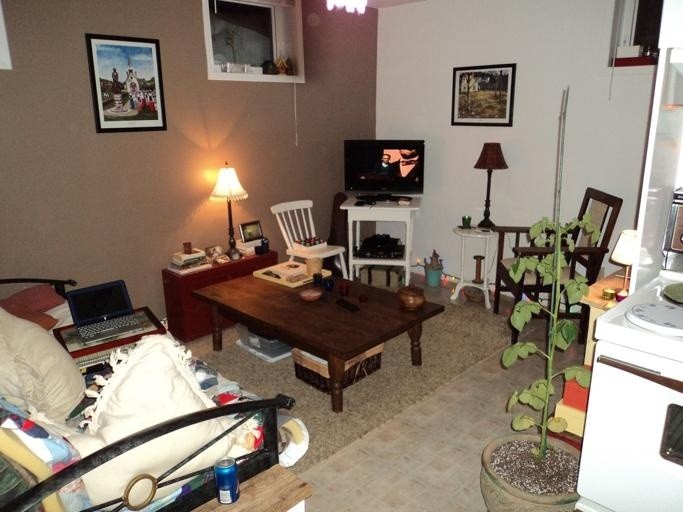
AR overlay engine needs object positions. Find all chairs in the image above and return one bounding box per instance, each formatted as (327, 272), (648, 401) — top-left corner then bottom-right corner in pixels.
(494, 187), (625, 348)
(271, 199), (350, 281)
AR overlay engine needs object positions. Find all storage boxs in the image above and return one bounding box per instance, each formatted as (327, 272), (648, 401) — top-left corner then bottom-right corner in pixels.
(233, 321), (294, 359)
(290, 343), (385, 394)
(359, 264), (404, 294)
(539, 366), (593, 449)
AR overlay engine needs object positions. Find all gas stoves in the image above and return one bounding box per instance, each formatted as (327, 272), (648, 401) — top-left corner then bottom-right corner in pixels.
(594, 271), (683, 336)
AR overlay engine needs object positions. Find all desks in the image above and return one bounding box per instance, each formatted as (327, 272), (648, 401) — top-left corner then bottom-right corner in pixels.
(448, 223), (504, 312)
(189, 463), (314, 511)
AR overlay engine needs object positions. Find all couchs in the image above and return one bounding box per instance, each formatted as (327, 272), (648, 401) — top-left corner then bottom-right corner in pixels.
(0, 277), (310, 511)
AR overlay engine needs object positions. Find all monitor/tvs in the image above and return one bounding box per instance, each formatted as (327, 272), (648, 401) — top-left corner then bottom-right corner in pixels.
(344, 140), (425, 202)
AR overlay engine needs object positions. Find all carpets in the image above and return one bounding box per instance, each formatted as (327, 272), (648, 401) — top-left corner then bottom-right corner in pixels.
(182, 282), (538, 475)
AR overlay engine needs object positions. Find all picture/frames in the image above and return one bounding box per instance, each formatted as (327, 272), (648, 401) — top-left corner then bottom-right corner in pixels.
(449, 62), (515, 128)
(238, 219), (264, 242)
(84, 32), (169, 135)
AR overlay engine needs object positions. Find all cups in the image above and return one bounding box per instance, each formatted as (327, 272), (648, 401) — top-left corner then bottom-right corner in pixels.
(183, 241), (191, 254)
(305, 255), (324, 276)
(461, 214), (471, 229)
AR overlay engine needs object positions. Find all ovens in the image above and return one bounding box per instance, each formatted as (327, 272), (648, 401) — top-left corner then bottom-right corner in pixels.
(569, 336), (683, 511)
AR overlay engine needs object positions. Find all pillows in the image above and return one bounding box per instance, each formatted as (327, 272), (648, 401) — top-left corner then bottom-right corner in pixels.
(1, 306), (88, 426)
(28, 332), (242, 508)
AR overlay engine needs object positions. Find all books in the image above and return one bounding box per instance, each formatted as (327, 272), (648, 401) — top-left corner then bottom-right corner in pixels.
(167, 246), (211, 277)
(253, 260), (331, 287)
(294, 236), (328, 252)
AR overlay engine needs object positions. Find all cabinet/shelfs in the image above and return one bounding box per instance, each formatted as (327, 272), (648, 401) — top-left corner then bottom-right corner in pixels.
(339, 195), (425, 289)
(161, 246), (278, 343)
(578, 267), (634, 363)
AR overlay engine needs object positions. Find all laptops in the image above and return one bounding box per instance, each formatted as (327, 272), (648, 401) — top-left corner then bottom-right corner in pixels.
(64, 280), (143, 344)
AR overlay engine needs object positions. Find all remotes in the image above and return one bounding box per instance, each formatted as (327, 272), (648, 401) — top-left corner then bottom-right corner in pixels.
(335, 299), (359, 313)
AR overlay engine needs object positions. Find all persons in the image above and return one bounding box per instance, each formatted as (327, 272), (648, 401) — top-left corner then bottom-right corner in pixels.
(378, 153), (392, 173)
(100, 67), (158, 116)
(388, 148), (420, 187)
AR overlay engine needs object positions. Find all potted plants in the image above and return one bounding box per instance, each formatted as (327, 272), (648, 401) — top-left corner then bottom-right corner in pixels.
(477, 208), (616, 511)
(424, 249), (444, 288)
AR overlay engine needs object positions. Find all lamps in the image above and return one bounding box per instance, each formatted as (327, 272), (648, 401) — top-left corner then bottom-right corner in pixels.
(609, 229), (635, 300)
(473, 141), (509, 229)
(209, 160), (250, 261)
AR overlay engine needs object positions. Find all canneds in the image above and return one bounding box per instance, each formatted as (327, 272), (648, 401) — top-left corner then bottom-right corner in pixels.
(213, 457), (241, 503)
